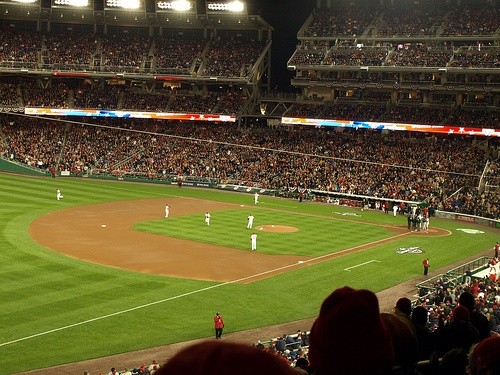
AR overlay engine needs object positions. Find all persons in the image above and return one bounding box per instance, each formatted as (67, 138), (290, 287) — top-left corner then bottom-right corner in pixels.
(83, 243), (500, 375)
(250, 233), (257, 251)
(0, 0), (500, 231)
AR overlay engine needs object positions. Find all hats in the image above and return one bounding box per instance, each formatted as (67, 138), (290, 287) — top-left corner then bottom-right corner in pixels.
(413, 306), (430, 314)
(279, 337), (283, 340)
(459, 292), (475, 305)
(308, 286), (394, 375)
(297, 329), (301, 332)
(396, 297), (411, 316)
(295, 358), (309, 367)
(380, 312), (418, 365)
(453, 306), (470, 322)
(216, 312), (219, 315)
